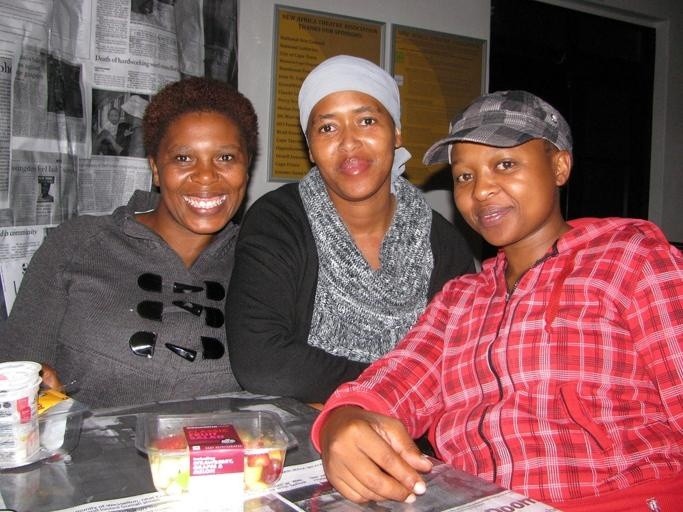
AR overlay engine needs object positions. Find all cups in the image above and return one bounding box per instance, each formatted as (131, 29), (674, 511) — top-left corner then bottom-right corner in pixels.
(0, 361), (43, 469)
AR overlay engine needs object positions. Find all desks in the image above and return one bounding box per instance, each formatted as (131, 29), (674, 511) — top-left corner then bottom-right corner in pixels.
(0, 391), (565, 512)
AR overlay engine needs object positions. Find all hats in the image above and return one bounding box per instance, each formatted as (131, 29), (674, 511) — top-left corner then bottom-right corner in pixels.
(422, 90), (574, 167)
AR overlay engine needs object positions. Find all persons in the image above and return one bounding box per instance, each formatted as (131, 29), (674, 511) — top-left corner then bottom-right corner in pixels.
(306, 86), (681, 511)
(223, 52), (478, 463)
(0, 74), (262, 412)
(36, 178), (54, 206)
(99, 107), (124, 146)
